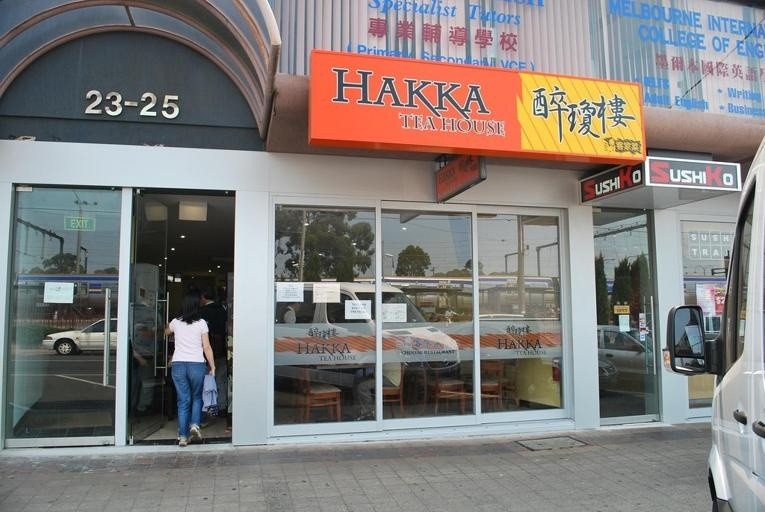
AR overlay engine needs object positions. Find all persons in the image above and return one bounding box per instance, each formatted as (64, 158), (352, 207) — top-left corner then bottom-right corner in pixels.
(191, 287), (229, 418)
(350, 361), (402, 420)
(163, 292), (216, 448)
(129, 290), (166, 415)
(274, 302), (302, 322)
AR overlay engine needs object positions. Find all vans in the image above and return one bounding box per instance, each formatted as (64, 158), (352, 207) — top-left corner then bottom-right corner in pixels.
(274, 281), (460, 404)
(665, 136), (764, 511)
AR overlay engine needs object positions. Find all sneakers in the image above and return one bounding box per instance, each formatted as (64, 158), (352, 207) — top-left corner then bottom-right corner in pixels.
(178, 411), (225, 447)
(355, 411), (375, 421)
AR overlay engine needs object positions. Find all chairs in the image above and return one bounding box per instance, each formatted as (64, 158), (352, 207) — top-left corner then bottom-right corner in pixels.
(293, 361), (505, 422)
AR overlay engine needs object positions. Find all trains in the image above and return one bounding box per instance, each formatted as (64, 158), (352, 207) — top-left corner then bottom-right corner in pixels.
(606, 276), (728, 305)
(12, 273), (119, 310)
(284, 275), (560, 297)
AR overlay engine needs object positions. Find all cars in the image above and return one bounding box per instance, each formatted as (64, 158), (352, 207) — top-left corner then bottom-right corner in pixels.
(41, 316), (118, 356)
(436, 309), (705, 391)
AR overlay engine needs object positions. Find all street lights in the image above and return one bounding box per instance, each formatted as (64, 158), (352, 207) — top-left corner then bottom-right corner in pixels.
(384, 253), (395, 276)
